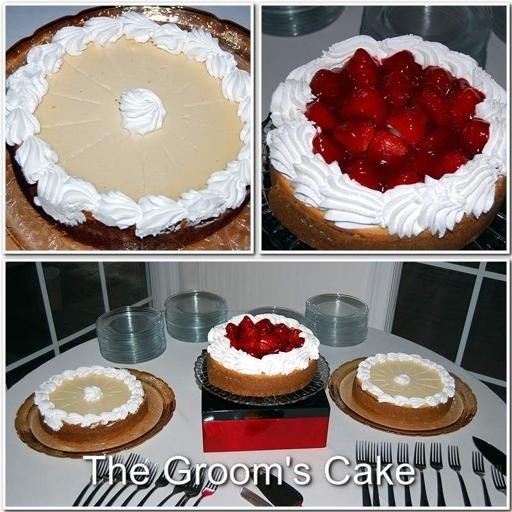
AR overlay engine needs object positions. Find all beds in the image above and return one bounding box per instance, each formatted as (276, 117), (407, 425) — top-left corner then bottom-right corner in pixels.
(6, 311), (507, 506)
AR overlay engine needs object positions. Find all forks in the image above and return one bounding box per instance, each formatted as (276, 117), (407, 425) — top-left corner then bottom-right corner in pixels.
(94, 453), (140, 506)
(104, 459), (151, 506)
(381, 442), (396, 506)
(368, 441), (379, 506)
(397, 442), (413, 506)
(413, 443), (429, 506)
(82, 453), (124, 506)
(180, 469), (205, 506)
(355, 440), (372, 506)
(471, 452), (491, 506)
(73, 459), (109, 506)
(137, 460), (179, 506)
(490, 465), (506, 496)
(448, 445), (471, 507)
(176, 470), (196, 506)
(157, 465), (195, 506)
(194, 468), (223, 507)
(122, 461), (159, 507)
(429, 443), (446, 506)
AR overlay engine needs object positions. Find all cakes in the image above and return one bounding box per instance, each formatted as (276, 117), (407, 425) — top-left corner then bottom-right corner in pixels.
(206, 313), (321, 397)
(33, 365), (149, 444)
(352, 352), (456, 425)
(265, 33), (506, 250)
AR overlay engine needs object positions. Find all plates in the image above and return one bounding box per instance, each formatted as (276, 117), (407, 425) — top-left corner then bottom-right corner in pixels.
(247, 306), (305, 328)
(263, 108), (505, 251)
(164, 291), (226, 340)
(304, 293), (368, 346)
(329, 356), (477, 436)
(14, 368), (176, 458)
(96, 305), (165, 362)
(194, 349), (330, 406)
(359, 8), (491, 69)
(488, 8), (506, 43)
(263, 6), (342, 36)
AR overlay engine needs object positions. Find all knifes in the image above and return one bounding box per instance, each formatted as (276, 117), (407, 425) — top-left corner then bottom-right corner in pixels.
(241, 488), (271, 506)
(250, 467), (303, 507)
(474, 436), (506, 476)
